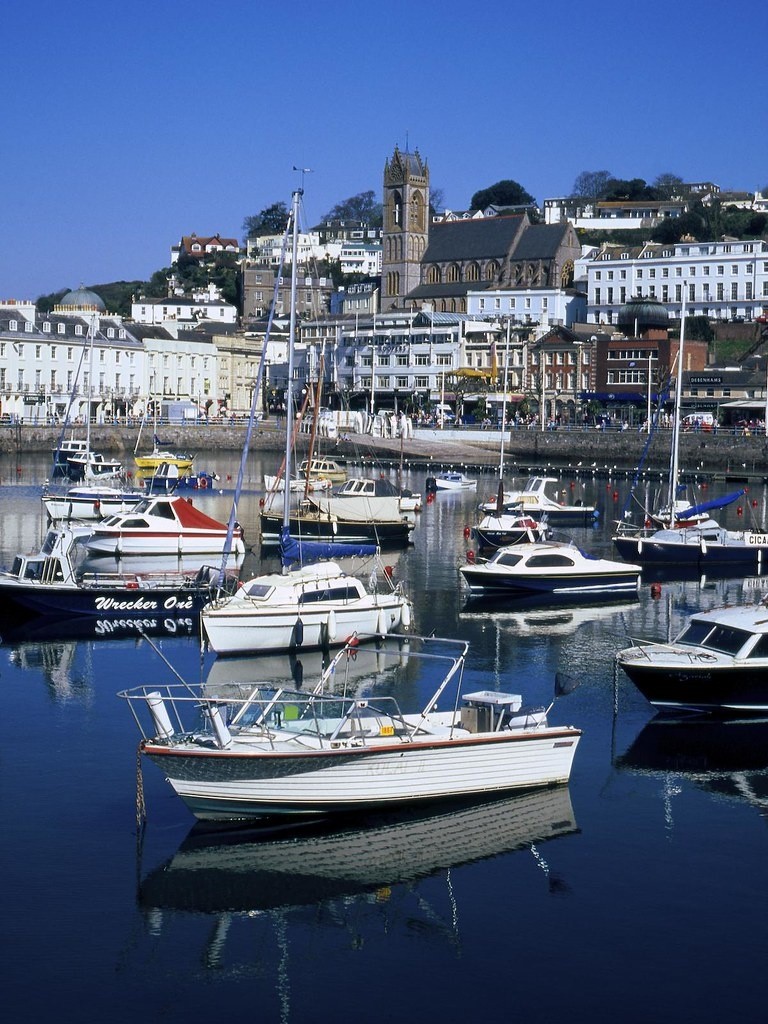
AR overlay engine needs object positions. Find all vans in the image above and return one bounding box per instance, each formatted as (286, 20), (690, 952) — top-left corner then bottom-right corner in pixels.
(682, 411), (714, 427)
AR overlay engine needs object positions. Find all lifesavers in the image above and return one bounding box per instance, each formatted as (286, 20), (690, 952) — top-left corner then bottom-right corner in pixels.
(489, 497), (495, 503)
(200, 477), (208, 488)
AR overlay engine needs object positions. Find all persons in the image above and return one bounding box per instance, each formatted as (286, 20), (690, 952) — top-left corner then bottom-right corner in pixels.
(482, 414), (556, 431)
(600, 413), (765, 435)
(412, 414), (462, 429)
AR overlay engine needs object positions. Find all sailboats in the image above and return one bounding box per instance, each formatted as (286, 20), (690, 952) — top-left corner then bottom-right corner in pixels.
(53, 311), (126, 483)
(609, 283), (768, 565)
(202, 189), (415, 656)
(257, 351), (415, 549)
(133, 369), (195, 470)
(264, 383), (333, 492)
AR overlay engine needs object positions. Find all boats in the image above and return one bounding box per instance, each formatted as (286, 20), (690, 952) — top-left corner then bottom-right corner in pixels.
(298, 457), (346, 481)
(458, 592), (640, 639)
(459, 529), (643, 597)
(463, 318), (556, 552)
(607, 591), (768, 716)
(135, 784), (580, 971)
(115, 630), (582, 821)
(430, 471), (478, 490)
(76, 493), (244, 556)
(328, 476), (423, 512)
(201, 630), (411, 716)
(41, 480), (146, 522)
(478, 475), (600, 528)
(142, 452), (222, 497)
(0, 516), (238, 622)
(612, 714), (768, 842)
